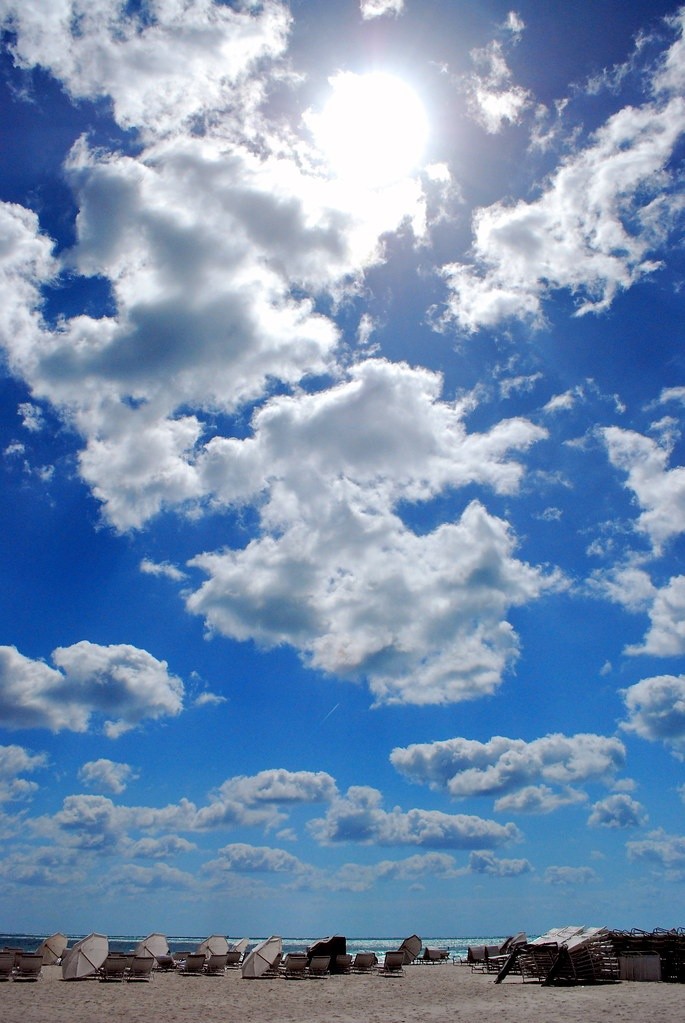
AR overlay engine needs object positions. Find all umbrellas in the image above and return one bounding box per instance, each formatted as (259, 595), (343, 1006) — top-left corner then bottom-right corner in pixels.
(35, 932), (68, 966)
(196, 935), (230, 972)
(230, 937), (249, 962)
(306, 935), (346, 974)
(397, 934), (426, 966)
(62, 932), (109, 982)
(134, 933), (170, 973)
(241, 935), (288, 979)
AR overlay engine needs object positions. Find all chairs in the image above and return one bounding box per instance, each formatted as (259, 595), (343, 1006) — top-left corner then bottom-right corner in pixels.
(0, 947), (408, 984)
(452, 926), (685, 987)
(417, 946), (450, 965)
